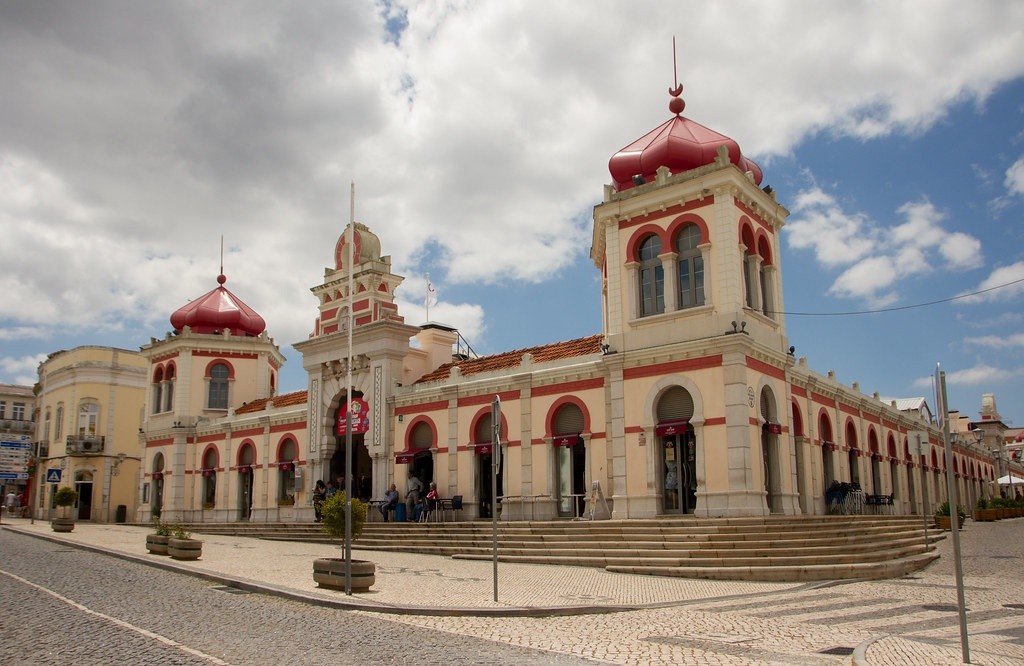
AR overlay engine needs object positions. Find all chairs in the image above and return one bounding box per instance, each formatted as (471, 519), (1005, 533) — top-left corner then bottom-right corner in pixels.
(386, 492), (398, 522)
(435, 496), (463, 523)
(418, 496), (441, 523)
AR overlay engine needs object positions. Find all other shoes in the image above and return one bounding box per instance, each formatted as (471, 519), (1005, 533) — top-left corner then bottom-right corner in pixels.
(408, 517), (419, 522)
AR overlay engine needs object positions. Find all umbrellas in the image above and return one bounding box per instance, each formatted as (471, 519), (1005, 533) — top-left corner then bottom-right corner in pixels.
(989, 475), (1024, 500)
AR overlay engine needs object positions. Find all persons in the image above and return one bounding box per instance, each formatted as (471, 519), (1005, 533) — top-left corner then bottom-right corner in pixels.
(1000, 486), (1022, 501)
(404, 471), (424, 521)
(378, 482), (399, 523)
(312, 480), (327, 523)
(16, 491), (24, 516)
(665, 457), (679, 510)
(324, 481), (336, 502)
(6, 490), (17, 517)
(666, 441), (673, 449)
(688, 440), (694, 461)
(335, 473), (372, 505)
(409, 482), (438, 523)
